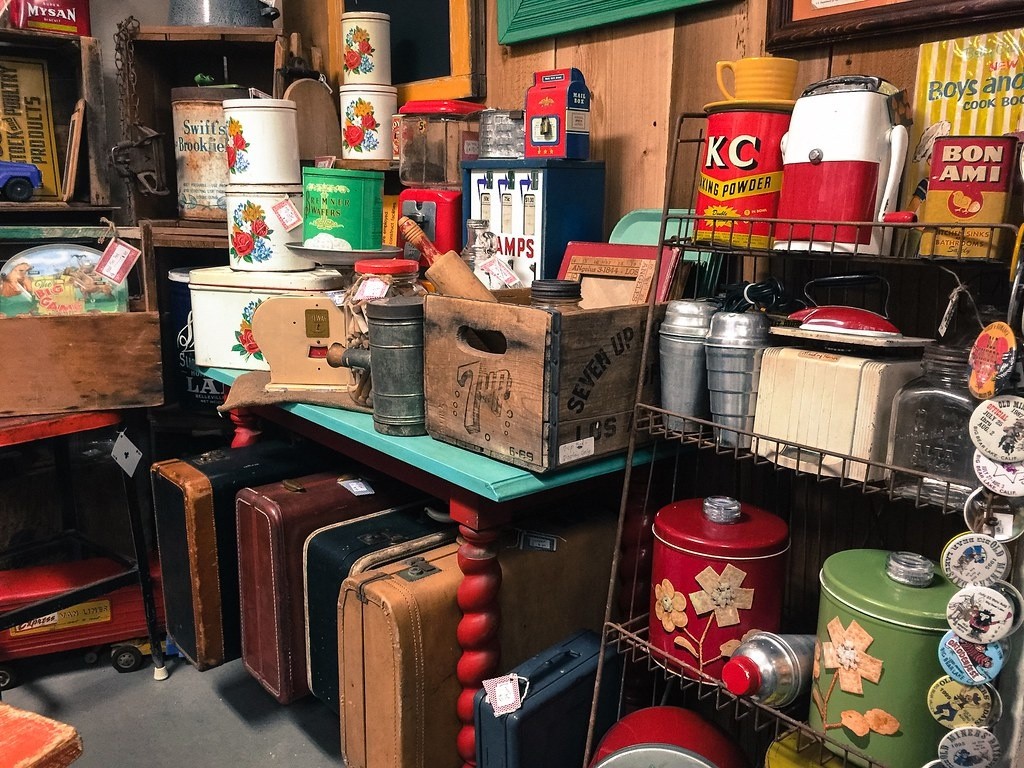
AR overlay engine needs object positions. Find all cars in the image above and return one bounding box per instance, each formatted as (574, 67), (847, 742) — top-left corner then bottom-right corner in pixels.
(0, 160), (44, 202)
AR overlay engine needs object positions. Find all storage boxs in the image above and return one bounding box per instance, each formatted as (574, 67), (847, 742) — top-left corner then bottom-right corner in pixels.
(422, 287), (659, 474)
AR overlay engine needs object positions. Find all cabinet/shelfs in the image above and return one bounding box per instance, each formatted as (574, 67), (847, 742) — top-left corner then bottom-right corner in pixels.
(581, 111), (1024, 768)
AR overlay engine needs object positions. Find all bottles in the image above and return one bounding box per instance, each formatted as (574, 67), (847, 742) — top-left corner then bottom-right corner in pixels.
(722, 630), (816, 710)
(527, 278), (584, 313)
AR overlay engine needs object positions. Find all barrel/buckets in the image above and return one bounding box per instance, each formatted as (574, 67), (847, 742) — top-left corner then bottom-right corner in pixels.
(703, 347), (765, 449)
(659, 334), (712, 432)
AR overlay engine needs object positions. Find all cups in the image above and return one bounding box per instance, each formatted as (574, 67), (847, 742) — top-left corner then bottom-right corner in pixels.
(716, 57), (800, 100)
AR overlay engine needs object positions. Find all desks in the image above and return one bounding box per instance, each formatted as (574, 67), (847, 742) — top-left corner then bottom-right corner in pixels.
(195, 367), (670, 768)
(0, 406), (170, 703)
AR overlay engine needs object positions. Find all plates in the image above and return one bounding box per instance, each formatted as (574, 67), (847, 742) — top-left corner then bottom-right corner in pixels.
(702, 101), (796, 111)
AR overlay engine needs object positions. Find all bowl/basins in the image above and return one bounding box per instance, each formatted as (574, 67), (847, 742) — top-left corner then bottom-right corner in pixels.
(658, 298), (721, 338)
(704, 310), (783, 348)
